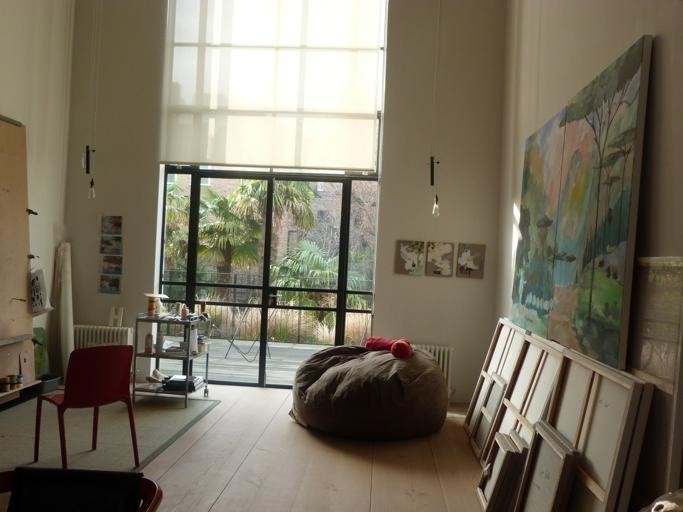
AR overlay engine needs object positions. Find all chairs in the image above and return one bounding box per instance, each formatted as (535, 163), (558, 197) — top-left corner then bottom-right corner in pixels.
(33, 345), (139, 471)
(0, 466), (162, 511)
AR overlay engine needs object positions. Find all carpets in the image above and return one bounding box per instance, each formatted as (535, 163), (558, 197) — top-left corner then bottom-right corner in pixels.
(0, 388), (221, 511)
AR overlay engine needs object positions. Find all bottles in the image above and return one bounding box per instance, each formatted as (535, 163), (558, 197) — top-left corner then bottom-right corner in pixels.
(177, 303), (181, 318)
(182, 304), (189, 320)
(147, 297), (155, 316)
(145, 333), (153, 354)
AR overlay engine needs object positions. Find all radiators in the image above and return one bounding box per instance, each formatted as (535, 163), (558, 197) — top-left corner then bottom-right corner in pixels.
(72, 324), (133, 351)
(410, 343), (456, 407)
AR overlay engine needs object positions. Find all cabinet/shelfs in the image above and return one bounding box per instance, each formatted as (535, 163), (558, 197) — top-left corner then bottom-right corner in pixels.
(131, 312), (210, 410)
